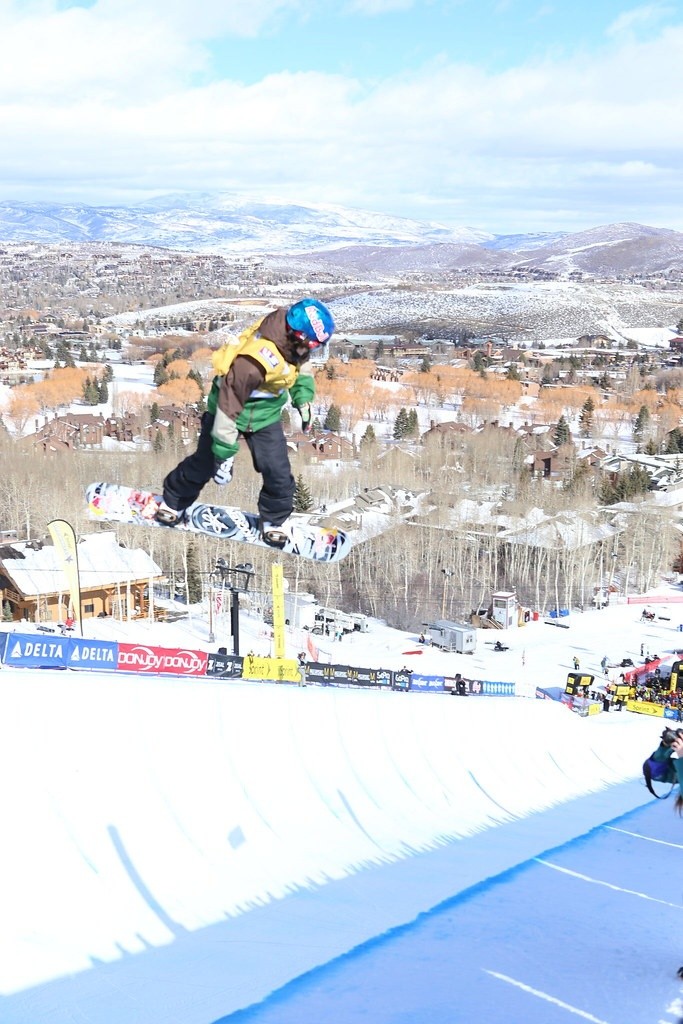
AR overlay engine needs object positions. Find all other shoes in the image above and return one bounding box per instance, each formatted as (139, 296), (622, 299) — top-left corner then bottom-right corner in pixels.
(154, 499), (184, 525)
(262, 518), (288, 547)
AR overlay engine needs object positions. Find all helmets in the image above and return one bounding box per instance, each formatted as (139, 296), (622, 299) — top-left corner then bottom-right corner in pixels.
(286, 298), (334, 344)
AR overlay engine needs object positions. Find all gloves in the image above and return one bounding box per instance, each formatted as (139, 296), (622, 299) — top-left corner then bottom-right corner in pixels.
(296, 403), (311, 433)
(213, 455), (234, 484)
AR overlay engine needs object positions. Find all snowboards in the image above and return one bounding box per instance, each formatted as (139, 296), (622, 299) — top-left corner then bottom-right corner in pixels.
(83, 481), (351, 563)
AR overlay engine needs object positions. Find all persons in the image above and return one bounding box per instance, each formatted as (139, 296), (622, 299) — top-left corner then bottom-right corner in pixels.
(601, 657), (608, 673)
(577, 689), (601, 701)
(640, 643), (644, 656)
(643, 732), (683, 816)
(573, 657), (580, 670)
(157, 299), (336, 547)
(401, 666), (413, 674)
(66, 617), (73, 628)
(640, 609), (647, 621)
(636, 674), (683, 723)
(418, 634), (425, 643)
(605, 684), (610, 693)
(645, 655), (660, 664)
(495, 641), (501, 648)
(297, 652), (309, 687)
(619, 672), (625, 680)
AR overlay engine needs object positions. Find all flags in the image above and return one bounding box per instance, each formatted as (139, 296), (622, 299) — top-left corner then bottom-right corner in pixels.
(48, 519), (81, 621)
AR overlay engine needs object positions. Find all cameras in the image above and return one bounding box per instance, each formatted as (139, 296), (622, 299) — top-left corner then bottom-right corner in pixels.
(660, 727), (683, 745)
